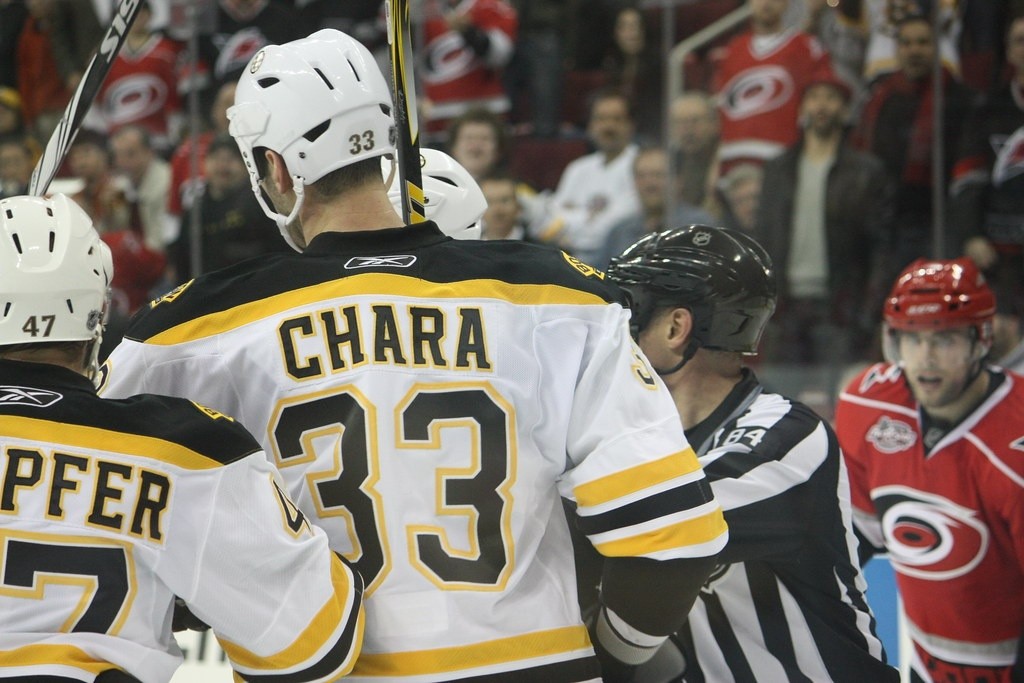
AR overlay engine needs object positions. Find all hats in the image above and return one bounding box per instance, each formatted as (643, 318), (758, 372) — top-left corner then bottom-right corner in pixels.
(803, 68), (856, 101)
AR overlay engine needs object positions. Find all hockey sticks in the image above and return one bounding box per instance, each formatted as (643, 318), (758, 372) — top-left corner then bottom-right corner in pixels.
(26, 0), (147, 195)
(384, 0), (429, 226)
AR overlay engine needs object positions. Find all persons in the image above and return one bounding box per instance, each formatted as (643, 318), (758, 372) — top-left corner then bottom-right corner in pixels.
(845, 18), (975, 264)
(835, 258), (1024, 683)
(0, 0), (1024, 374)
(479, 178), (546, 244)
(0, 194), (364, 683)
(555, 97), (642, 262)
(564, 225), (901, 683)
(598, 148), (718, 266)
(757, 72), (901, 365)
(88, 26), (729, 683)
(714, 0), (830, 174)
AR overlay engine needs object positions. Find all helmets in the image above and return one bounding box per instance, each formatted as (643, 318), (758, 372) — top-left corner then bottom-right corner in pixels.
(0, 192), (116, 345)
(225, 28), (398, 227)
(380, 146), (489, 240)
(884, 255), (998, 344)
(606, 223), (781, 356)
